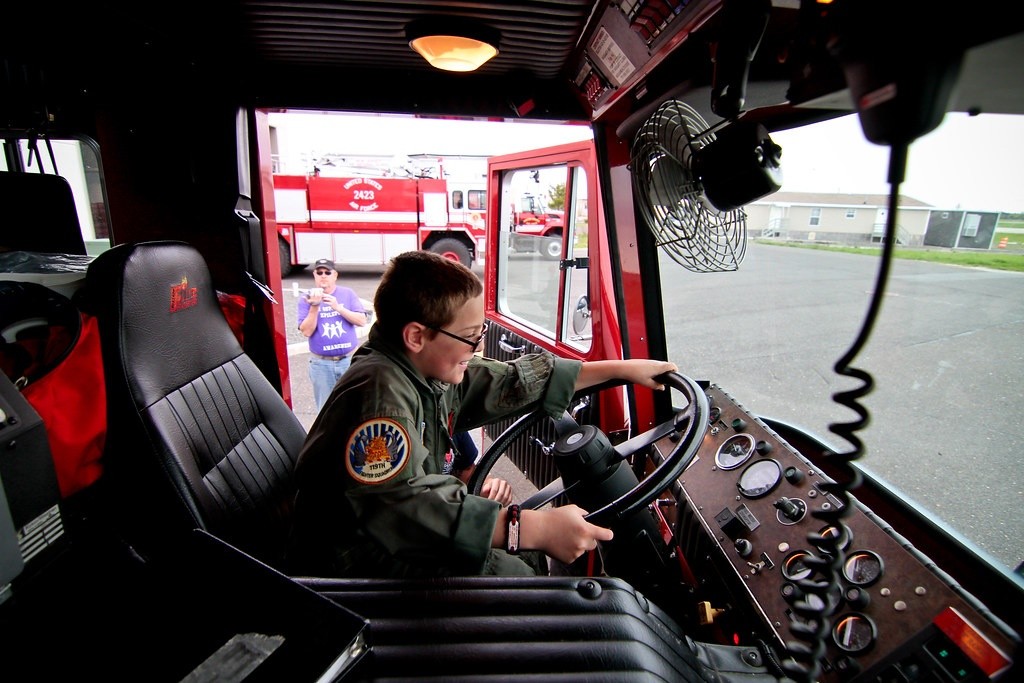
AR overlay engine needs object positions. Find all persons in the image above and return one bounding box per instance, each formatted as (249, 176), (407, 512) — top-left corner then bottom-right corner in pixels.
(294, 251), (678, 577)
(296, 259), (368, 413)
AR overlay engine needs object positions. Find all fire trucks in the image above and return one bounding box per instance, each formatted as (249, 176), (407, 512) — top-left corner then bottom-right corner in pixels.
(272, 151), (572, 277)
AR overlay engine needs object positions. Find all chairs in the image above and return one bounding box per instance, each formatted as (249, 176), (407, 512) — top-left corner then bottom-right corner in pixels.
(86, 238), (546, 577)
(0, 170), (87, 256)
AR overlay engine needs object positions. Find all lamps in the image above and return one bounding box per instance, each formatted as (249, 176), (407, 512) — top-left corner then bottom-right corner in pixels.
(405, 16), (504, 71)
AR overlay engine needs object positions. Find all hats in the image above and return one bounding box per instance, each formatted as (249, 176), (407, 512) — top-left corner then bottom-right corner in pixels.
(304, 259), (337, 270)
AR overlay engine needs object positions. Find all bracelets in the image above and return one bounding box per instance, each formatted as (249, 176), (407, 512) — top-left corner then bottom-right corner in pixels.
(506, 505), (520, 554)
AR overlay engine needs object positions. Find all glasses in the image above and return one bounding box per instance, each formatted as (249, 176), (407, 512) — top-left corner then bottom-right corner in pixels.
(427, 323), (488, 352)
(316, 271), (331, 275)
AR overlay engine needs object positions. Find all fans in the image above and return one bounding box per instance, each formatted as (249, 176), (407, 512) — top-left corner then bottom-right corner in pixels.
(629, 0), (786, 271)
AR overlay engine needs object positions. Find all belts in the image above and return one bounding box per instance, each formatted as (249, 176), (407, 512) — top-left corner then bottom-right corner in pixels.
(311, 352), (346, 360)
